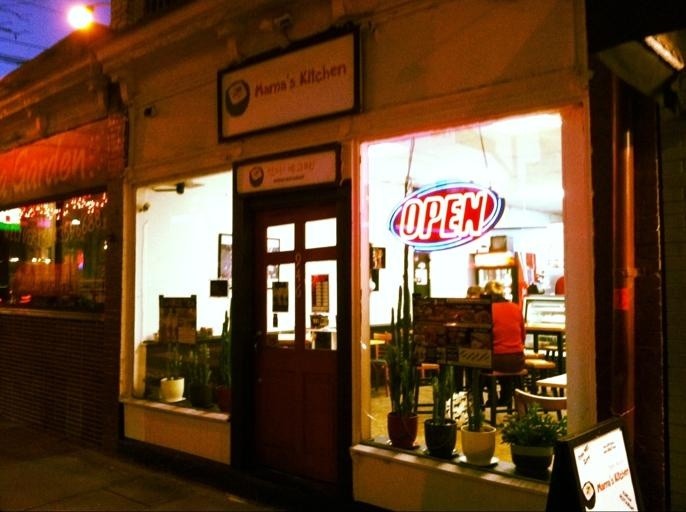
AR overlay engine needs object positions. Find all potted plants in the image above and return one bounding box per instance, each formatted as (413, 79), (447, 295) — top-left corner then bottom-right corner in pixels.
(381, 287), (420, 448)
(501, 398), (567, 476)
(147, 311), (236, 411)
(424, 354), (456, 458)
(457, 364), (499, 468)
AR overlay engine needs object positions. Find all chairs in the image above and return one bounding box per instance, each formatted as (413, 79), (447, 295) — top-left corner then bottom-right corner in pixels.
(417, 364), (440, 387)
(374, 333), (394, 398)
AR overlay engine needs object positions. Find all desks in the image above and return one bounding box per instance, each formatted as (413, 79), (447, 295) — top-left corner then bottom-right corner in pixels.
(370, 340), (386, 391)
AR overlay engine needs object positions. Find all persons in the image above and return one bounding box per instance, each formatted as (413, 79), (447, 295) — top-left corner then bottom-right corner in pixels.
(528, 274), (545, 293)
(467, 280), (526, 406)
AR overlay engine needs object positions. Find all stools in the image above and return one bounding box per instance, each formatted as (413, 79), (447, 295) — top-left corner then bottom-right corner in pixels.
(436, 340), (560, 427)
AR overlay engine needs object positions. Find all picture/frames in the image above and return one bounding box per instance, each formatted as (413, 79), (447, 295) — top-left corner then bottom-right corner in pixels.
(217, 233), (282, 291)
